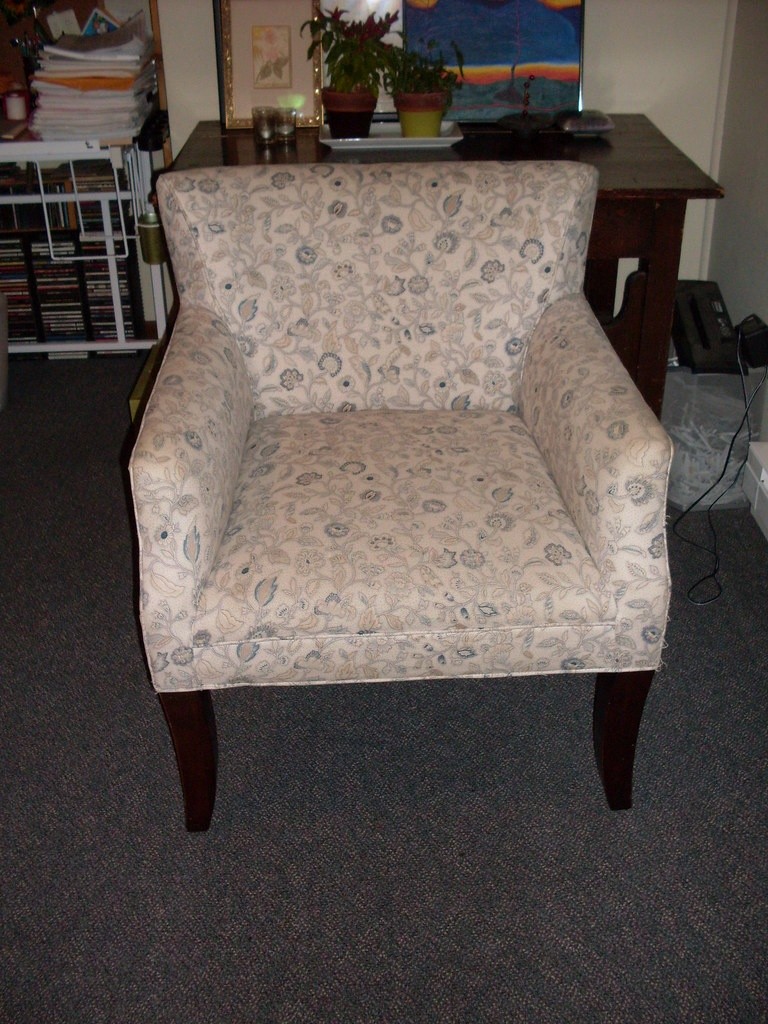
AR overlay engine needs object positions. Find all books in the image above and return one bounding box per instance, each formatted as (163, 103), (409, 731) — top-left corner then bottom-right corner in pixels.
(1, 165), (146, 360)
(30, 10), (156, 141)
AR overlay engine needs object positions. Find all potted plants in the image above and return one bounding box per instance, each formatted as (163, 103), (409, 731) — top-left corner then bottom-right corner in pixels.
(299, 7), (398, 140)
(382, 33), (464, 136)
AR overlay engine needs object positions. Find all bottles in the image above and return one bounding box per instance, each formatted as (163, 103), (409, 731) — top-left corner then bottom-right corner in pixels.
(138, 212), (168, 265)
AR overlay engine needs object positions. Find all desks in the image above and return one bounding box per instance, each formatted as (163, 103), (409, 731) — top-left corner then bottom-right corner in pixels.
(148, 113), (724, 421)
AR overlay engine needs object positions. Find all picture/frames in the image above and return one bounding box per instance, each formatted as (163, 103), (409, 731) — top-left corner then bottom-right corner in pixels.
(402, 0), (586, 124)
(220, 0), (324, 130)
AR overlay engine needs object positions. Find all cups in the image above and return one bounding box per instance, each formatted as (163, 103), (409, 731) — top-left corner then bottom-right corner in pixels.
(275, 108), (296, 141)
(5, 95), (26, 121)
(251, 105), (276, 144)
(21, 57), (41, 91)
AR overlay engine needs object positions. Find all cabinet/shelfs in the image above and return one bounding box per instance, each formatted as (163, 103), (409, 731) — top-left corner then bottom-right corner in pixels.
(0, 140), (168, 356)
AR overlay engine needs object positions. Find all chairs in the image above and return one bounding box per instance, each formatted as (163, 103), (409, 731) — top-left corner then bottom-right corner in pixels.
(128, 161), (675, 808)
